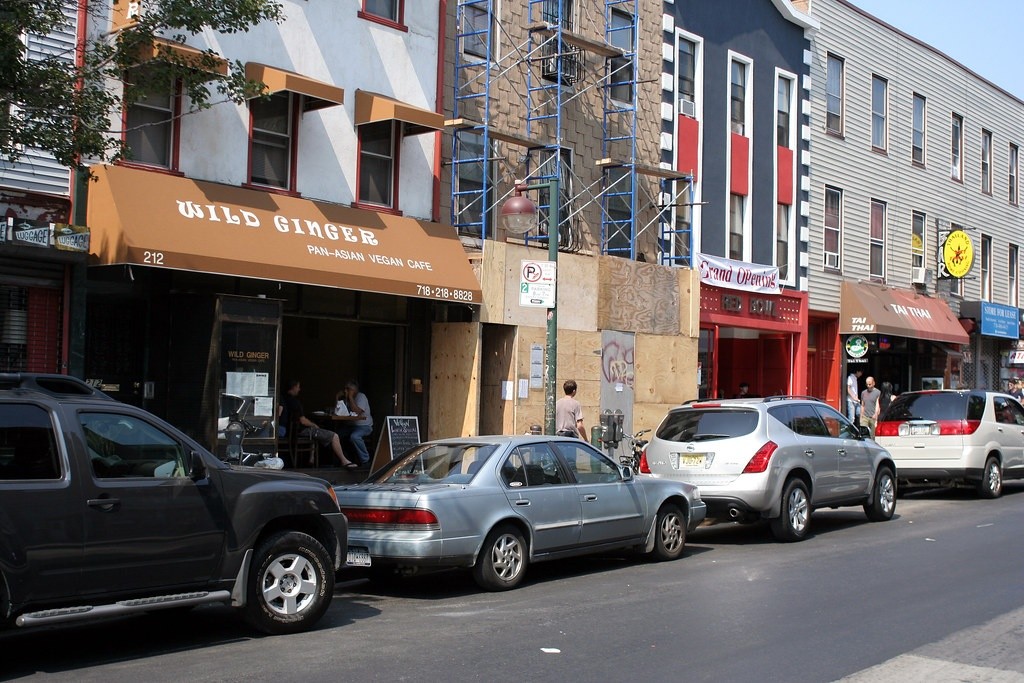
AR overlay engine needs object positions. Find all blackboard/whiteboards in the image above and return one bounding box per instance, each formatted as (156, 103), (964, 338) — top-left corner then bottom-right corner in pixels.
(368, 415), (426, 477)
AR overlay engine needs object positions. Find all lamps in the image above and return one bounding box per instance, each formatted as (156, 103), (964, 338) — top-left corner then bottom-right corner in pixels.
(413, 379), (423, 392)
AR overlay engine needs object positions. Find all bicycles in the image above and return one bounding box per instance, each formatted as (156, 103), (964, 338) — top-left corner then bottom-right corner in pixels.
(619, 430), (652, 471)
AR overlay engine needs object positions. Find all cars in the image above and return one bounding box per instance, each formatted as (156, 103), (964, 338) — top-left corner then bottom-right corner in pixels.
(330, 433), (706, 593)
(875, 389), (1024, 498)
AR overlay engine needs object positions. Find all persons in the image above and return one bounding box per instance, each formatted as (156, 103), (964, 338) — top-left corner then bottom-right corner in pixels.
(737, 383), (750, 398)
(279, 377), (358, 470)
(556, 378), (590, 472)
(334, 378), (374, 468)
(1007, 380), (1024, 407)
(847, 367), (897, 439)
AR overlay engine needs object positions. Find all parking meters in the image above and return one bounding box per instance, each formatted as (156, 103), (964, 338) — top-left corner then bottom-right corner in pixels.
(599, 408), (625, 472)
(224, 414), (244, 466)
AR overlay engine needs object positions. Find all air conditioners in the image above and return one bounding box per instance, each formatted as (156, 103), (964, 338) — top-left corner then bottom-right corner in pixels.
(912, 266), (933, 285)
(547, 47), (578, 79)
(678, 98), (695, 117)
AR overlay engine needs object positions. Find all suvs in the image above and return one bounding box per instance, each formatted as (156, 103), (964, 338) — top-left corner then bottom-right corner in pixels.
(0, 370), (349, 665)
(637, 396), (898, 542)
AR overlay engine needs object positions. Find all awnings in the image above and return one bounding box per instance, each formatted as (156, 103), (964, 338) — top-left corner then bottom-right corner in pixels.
(839, 279), (970, 345)
(70, 156), (482, 305)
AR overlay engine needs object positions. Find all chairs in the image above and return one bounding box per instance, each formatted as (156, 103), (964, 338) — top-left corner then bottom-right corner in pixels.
(508, 464), (546, 485)
(295, 421), (320, 468)
(277, 422), (294, 465)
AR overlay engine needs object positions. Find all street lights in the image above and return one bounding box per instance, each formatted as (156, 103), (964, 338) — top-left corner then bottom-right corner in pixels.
(503, 176), (559, 434)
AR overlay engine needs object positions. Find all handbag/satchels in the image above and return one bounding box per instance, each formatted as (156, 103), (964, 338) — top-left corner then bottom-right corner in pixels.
(854, 401), (861, 417)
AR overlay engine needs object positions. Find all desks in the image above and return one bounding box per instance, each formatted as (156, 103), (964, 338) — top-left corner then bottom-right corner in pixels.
(311, 412), (366, 467)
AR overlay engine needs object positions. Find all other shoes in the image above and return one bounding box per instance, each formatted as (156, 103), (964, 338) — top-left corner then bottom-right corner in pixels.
(344, 462), (358, 469)
